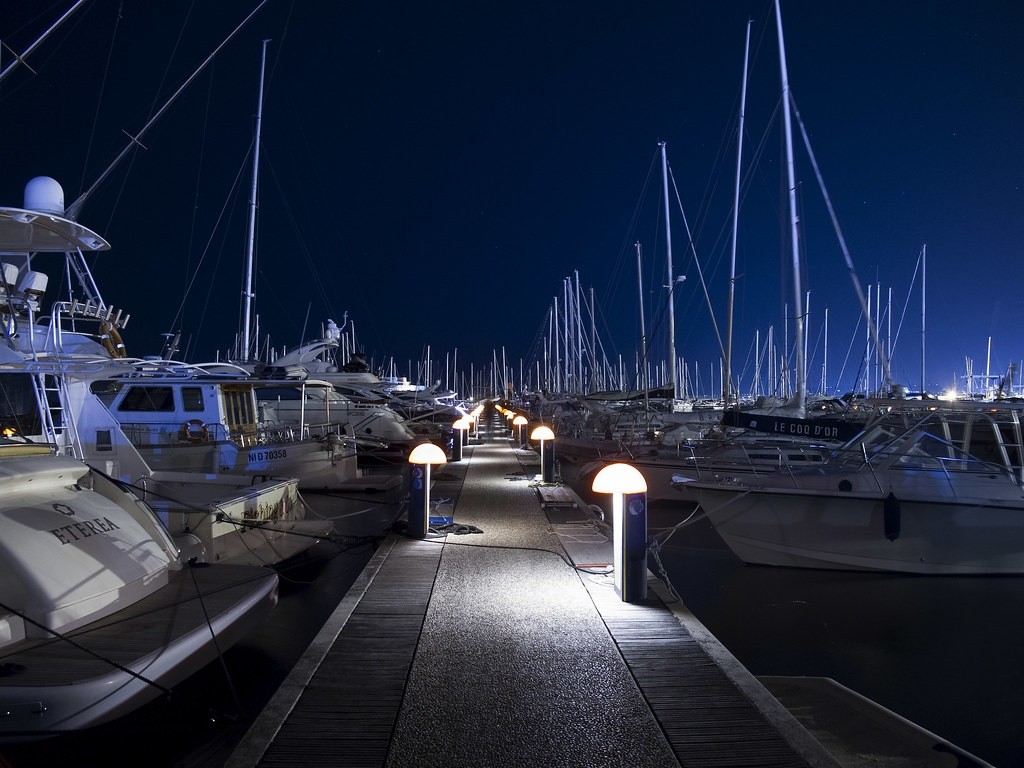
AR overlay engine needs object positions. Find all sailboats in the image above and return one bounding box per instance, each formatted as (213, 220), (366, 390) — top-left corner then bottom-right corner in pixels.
(1, 0), (1023, 734)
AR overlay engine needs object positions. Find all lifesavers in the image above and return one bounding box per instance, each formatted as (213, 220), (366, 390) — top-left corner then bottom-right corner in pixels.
(184, 419), (209, 444)
